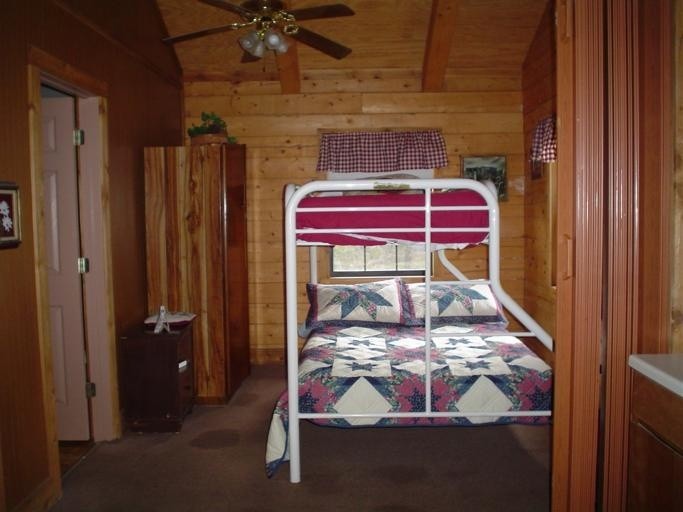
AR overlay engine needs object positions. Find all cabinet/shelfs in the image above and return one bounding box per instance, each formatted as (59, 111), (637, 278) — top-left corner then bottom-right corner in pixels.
(625, 368), (683, 512)
(117, 322), (194, 434)
(143, 143), (251, 405)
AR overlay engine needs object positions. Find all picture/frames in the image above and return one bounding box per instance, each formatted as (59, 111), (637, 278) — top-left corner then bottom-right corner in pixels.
(0, 181), (22, 249)
(460, 154), (508, 202)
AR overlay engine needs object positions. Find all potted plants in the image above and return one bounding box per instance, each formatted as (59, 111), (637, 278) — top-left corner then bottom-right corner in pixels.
(187, 110), (237, 144)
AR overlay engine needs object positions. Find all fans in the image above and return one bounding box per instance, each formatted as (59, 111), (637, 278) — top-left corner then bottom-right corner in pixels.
(161, 0), (356, 60)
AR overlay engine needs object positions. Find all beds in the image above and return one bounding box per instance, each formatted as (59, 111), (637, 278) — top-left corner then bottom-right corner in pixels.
(284, 179), (555, 485)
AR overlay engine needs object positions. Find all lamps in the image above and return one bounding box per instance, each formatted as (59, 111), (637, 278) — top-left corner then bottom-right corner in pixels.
(238, 18), (289, 59)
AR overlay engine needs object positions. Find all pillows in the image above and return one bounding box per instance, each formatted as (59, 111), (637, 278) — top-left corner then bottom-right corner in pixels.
(298, 279), (510, 339)
